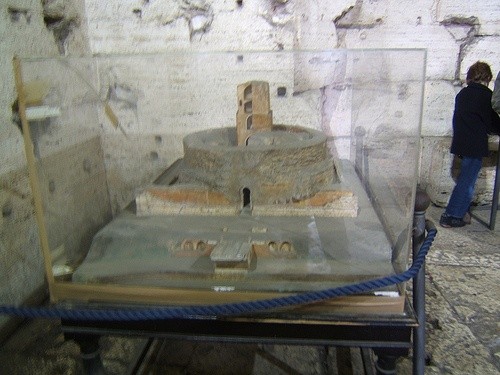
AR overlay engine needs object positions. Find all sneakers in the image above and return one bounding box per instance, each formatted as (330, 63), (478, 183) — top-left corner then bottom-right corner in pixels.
(439, 213), (466, 228)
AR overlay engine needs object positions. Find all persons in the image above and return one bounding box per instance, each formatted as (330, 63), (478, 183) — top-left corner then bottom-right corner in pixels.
(438, 61), (499, 229)
